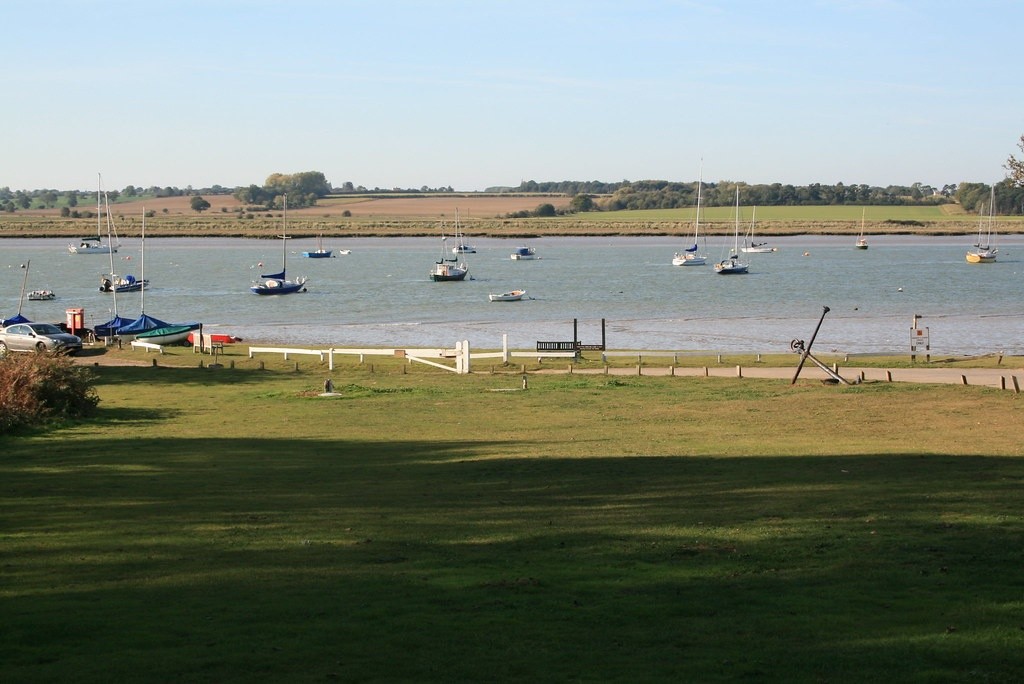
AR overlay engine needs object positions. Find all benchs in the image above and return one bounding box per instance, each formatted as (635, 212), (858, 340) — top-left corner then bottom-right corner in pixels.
(193, 333), (224, 356)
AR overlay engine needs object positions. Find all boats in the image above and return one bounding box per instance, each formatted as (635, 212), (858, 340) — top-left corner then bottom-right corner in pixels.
(98, 274), (150, 293)
(302, 231), (333, 259)
(452, 245), (477, 253)
(26, 290), (56, 301)
(188, 331), (244, 344)
(136, 322), (205, 346)
(511, 246), (536, 260)
(488, 289), (525, 302)
(340, 249), (352, 255)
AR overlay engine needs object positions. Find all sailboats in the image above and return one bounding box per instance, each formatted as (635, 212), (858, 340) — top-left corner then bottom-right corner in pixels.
(966, 183), (998, 264)
(429, 207), (469, 282)
(740, 205), (777, 253)
(1, 258), (32, 328)
(68, 173), (122, 254)
(250, 192), (310, 296)
(672, 157), (708, 266)
(116, 207), (170, 342)
(855, 207), (869, 250)
(93, 193), (138, 342)
(713, 185), (749, 275)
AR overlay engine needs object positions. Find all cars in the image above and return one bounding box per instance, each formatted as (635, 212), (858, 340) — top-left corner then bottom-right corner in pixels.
(0, 323), (83, 360)
